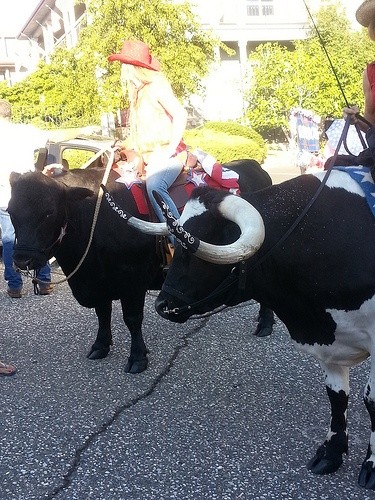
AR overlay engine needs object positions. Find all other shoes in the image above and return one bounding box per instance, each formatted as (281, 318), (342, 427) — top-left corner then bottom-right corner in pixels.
(39, 280), (55, 294)
(8, 289), (21, 297)
(0, 360), (17, 376)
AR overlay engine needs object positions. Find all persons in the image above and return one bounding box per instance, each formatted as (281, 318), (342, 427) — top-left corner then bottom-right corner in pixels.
(0, 102), (98, 375)
(107, 40), (188, 272)
(288, 1), (375, 178)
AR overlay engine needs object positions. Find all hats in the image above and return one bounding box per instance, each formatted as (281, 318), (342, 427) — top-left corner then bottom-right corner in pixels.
(108, 40), (161, 71)
(355, 0), (375, 27)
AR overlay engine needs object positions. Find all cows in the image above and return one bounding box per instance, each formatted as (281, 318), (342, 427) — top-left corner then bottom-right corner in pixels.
(155, 166), (374, 492)
(8, 159), (275, 374)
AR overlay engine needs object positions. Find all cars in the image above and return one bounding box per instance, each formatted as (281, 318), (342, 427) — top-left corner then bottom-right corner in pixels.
(34, 139), (116, 170)
